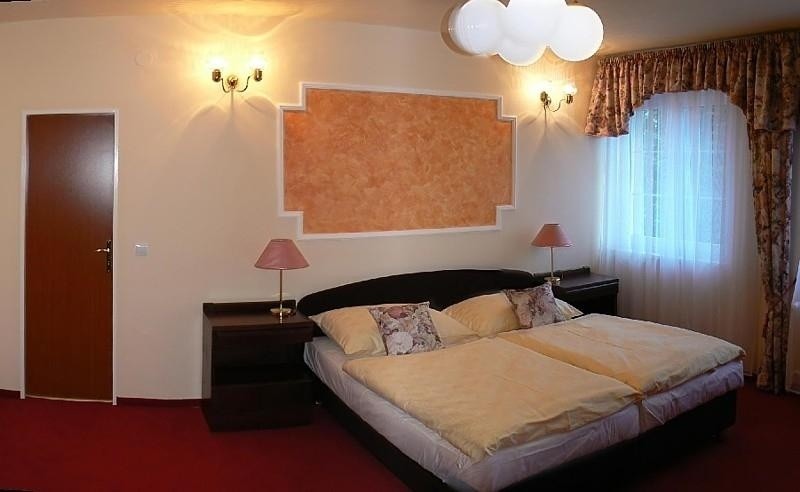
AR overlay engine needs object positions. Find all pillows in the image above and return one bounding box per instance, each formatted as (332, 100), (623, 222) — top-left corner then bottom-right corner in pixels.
(439, 289), (587, 340)
(504, 280), (570, 329)
(366, 299), (447, 358)
(304, 302), (477, 362)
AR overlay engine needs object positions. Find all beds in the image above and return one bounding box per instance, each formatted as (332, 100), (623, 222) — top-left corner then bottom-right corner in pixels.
(295, 264), (747, 492)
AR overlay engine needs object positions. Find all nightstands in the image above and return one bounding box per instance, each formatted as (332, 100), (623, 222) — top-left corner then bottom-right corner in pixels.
(533, 266), (624, 320)
(197, 295), (319, 435)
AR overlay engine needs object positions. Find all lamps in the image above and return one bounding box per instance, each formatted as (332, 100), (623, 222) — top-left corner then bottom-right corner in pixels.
(252, 237), (311, 318)
(530, 221), (571, 284)
(212, 60), (264, 97)
(534, 80), (579, 116)
(433, 1), (608, 68)
